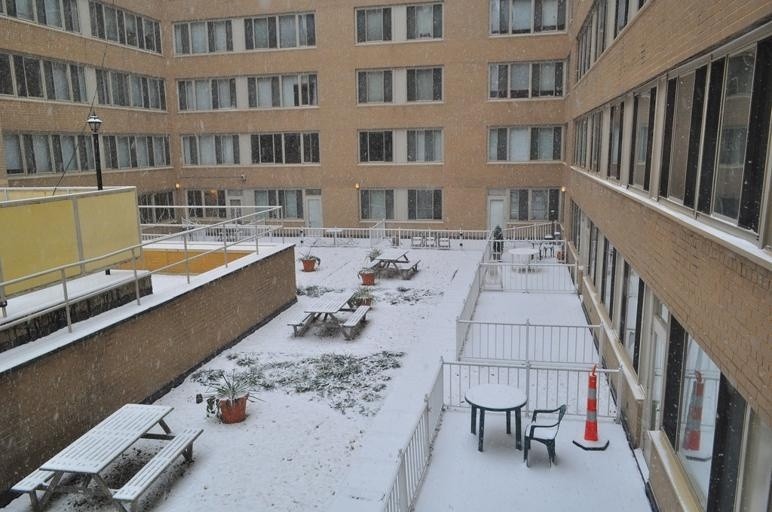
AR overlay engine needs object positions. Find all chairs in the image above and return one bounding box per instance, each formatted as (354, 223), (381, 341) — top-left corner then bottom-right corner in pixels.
(524, 404), (566, 470)
(544, 235), (554, 258)
(531, 242), (542, 260)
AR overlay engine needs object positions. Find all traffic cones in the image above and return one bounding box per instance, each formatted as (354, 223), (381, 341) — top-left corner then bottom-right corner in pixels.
(574, 366), (609, 451)
(681, 370), (712, 461)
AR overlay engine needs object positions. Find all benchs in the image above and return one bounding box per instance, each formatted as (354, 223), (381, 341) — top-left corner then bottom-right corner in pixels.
(363, 259), (421, 280)
(287, 305), (371, 340)
(12, 426), (204, 512)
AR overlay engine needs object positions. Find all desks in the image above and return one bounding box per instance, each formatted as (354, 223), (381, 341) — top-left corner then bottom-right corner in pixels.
(465, 384), (528, 452)
(510, 248), (539, 271)
(299, 292), (365, 339)
(374, 249), (418, 279)
(39, 404), (192, 512)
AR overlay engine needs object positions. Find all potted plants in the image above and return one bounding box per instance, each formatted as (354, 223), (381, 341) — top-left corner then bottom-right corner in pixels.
(195, 370), (263, 424)
(356, 262), (379, 285)
(295, 239), (321, 271)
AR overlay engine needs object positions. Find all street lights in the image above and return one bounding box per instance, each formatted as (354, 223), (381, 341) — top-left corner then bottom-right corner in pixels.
(88, 110), (113, 276)
